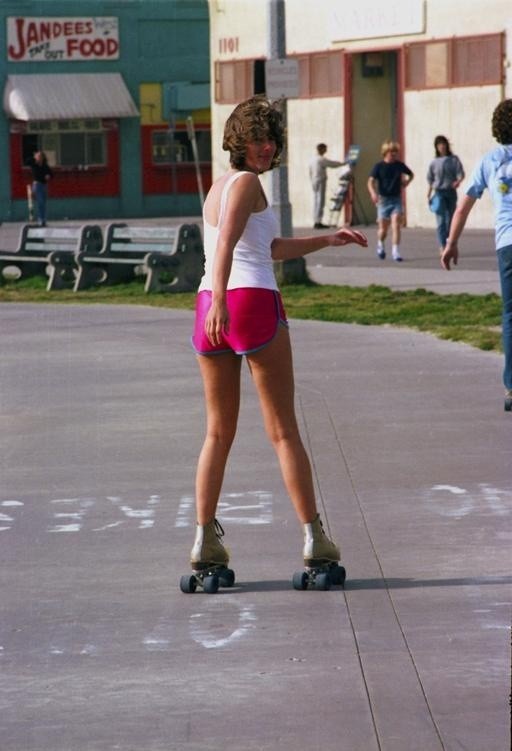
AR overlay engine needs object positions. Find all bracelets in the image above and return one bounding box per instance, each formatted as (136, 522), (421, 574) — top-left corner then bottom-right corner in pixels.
(445, 238), (457, 245)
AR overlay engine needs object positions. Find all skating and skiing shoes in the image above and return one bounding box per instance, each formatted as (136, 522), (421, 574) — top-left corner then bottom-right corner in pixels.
(292, 513), (346, 590)
(180, 518), (234, 594)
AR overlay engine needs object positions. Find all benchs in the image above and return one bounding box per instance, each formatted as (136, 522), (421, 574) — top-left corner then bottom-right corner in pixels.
(72, 222), (206, 293)
(0, 223), (102, 290)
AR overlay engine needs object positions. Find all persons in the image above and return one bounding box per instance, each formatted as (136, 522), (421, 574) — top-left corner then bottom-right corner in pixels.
(367, 140), (413, 262)
(188, 95), (368, 573)
(309, 142), (348, 230)
(31, 151), (52, 225)
(439, 97), (512, 410)
(427, 135), (464, 269)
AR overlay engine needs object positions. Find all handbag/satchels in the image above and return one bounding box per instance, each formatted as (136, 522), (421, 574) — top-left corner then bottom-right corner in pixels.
(429, 192), (445, 214)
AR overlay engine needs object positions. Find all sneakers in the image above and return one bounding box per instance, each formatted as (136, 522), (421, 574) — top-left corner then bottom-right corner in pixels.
(504, 388), (512, 412)
(392, 253), (401, 262)
(377, 249), (385, 259)
(313, 223), (329, 229)
